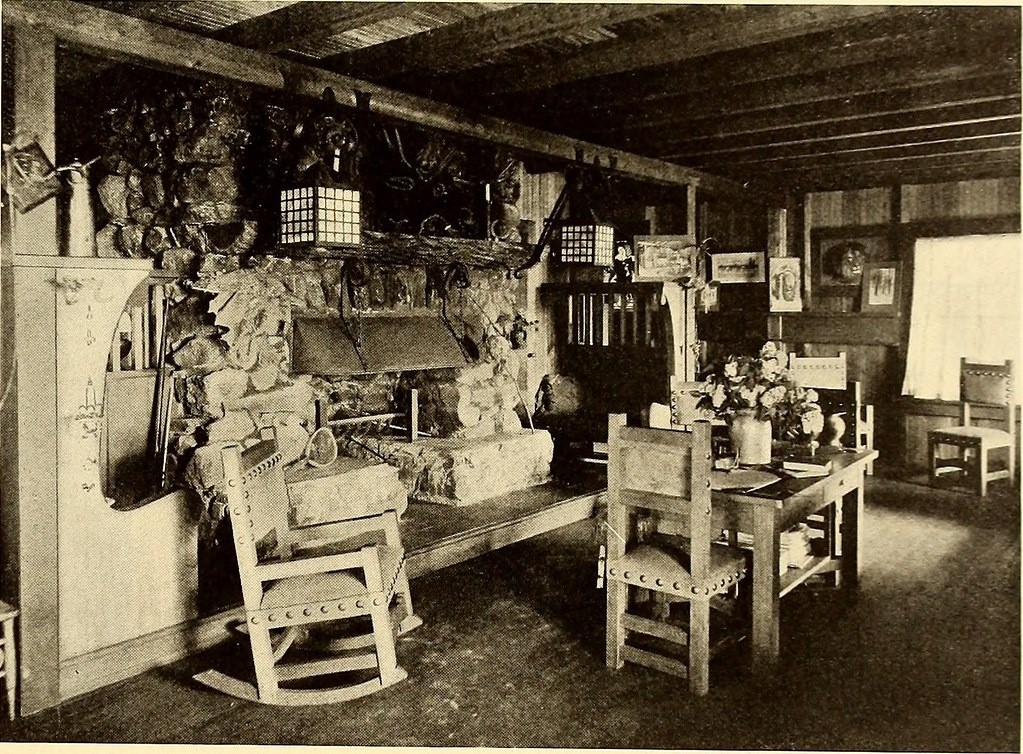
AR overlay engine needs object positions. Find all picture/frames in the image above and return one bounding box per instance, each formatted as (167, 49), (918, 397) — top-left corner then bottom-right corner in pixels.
(858, 259), (905, 321)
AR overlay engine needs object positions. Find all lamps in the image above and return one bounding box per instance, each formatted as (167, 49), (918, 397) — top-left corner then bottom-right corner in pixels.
(557, 168), (616, 268)
(276, 105), (364, 252)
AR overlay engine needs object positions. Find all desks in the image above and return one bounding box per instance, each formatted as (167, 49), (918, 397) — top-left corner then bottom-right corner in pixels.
(595, 431), (881, 665)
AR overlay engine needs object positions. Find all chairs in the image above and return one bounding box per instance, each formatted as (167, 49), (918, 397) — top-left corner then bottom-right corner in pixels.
(784, 349), (876, 589)
(602, 406), (755, 697)
(192, 426), (425, 709)
(925, 354), (1018, 501)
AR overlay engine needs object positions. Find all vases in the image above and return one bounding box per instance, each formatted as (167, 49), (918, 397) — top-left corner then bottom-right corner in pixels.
(728, 417), (774, 469)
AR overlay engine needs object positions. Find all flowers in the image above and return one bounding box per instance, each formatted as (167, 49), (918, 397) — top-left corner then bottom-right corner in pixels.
(686, 339), (825, 435)
